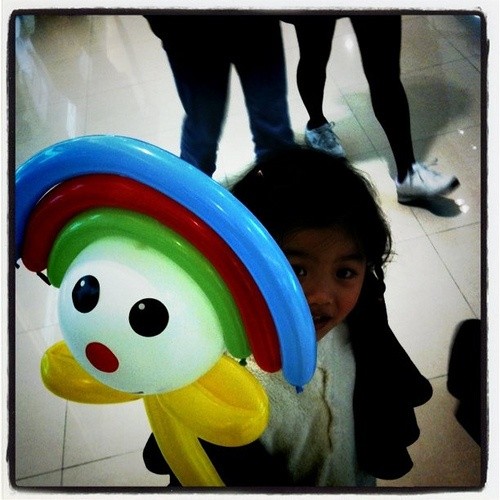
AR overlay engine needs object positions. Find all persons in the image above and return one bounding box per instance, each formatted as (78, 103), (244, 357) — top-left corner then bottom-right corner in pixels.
(141, 147), (433, 489)
(276, 10), (463, 199)
(143, 14), (295, 180)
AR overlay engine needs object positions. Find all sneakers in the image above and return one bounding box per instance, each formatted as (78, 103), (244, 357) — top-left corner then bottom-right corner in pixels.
(303, 119), (345, 160)
(395, 163), (459, 203)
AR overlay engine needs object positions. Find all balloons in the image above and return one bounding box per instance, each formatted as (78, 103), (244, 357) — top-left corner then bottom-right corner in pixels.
(15, 133), (319, 489)
